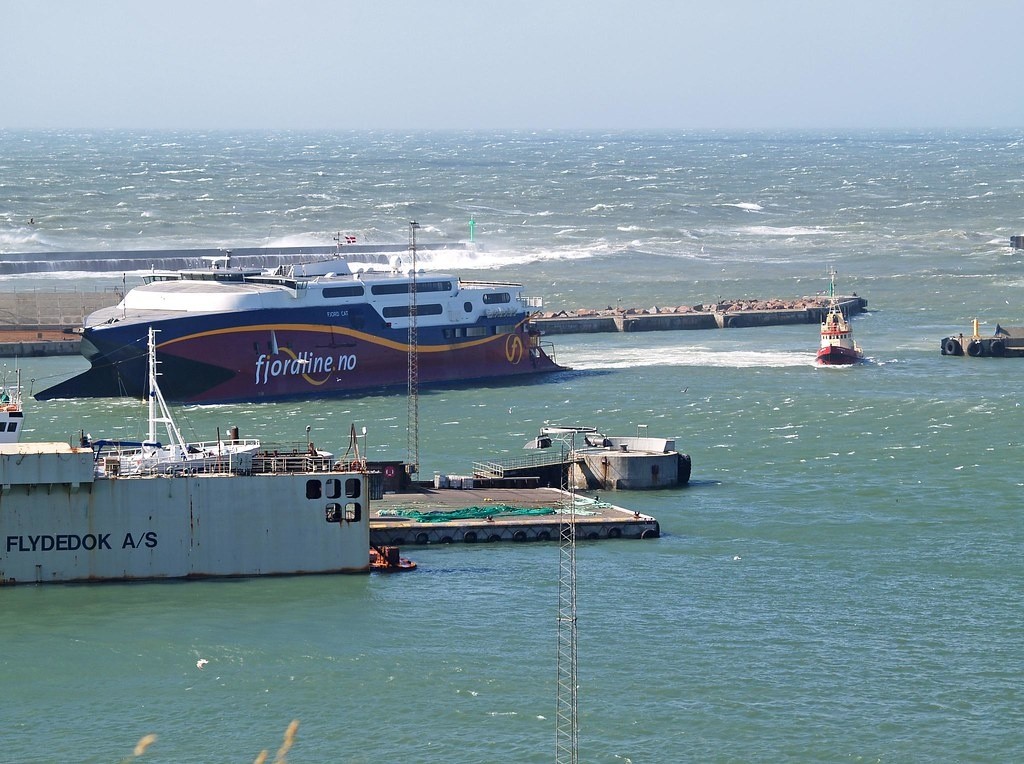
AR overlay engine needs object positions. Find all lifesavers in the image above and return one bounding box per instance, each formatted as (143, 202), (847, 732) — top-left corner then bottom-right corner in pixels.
(990, 342), (1003, 355)
(945, 340), (958, 355)
(391, 526), (658, 546)
(728, 318), (737, 328)
(386, 467), (395, 477)
(967, 342), (983, 357)
(351, 460), (361, 471)
(628, 321), (639, 332)
(333, 461), (343, 470)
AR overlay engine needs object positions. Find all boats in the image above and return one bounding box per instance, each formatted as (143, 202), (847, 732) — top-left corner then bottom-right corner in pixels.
(814, 265), (865, 366)
(79, 220), (573, 405)
